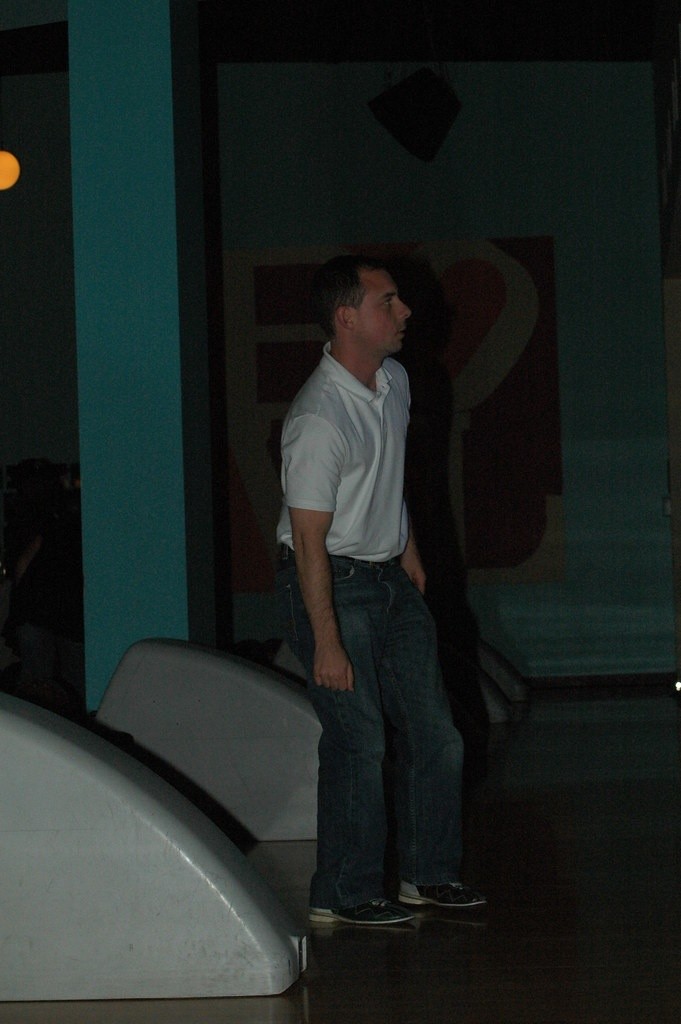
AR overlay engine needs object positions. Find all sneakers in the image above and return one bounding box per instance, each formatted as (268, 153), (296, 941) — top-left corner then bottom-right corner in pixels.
(309, 895), (414, 924)
(398, 881), (487, 907)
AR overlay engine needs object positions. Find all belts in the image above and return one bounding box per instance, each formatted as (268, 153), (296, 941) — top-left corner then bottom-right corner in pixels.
(328, 554), (402, 569)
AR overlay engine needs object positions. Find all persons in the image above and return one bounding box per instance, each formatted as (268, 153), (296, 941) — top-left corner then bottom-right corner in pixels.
(0, 459), (78, 665)
(276, 257), (488, 927)
